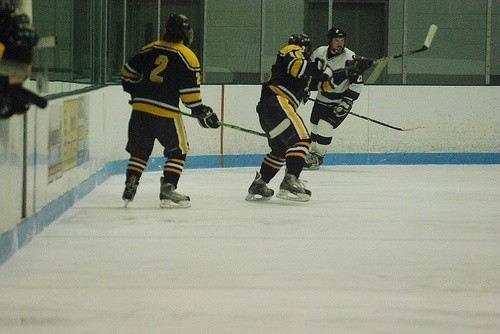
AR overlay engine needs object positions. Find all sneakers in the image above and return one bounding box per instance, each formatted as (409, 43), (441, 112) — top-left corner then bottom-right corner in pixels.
(304, 151), (325, 170)
(244, 170), (275, 202)
(275, 166), (312, 201)
(122, 172), (140, 208)
(159, 176), (191, 208)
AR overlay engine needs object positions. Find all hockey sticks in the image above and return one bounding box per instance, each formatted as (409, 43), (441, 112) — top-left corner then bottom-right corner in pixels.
(180, 111), (291, 139)
(333, 23), (438, 74)
(307, 95), (426, 132)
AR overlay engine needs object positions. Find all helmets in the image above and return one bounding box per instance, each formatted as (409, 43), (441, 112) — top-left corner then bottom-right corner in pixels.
(288, 33), (312, 60)
(165, 13), (193, 46)
(327, 27), (346, 40)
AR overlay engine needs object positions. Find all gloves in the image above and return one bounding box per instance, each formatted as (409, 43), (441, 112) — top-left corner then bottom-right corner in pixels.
(300, 86), (311, 106)
(345, 55), (374, 83)
(313, 56), (334, 81)
(192, 105), (221, 128)
(332, 96), (354, 118)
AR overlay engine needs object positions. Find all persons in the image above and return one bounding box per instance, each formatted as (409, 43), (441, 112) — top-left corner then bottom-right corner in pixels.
(121, 14), (221, 208)
(302, 28), (363, 170)
(246, 34), (332, 202)
